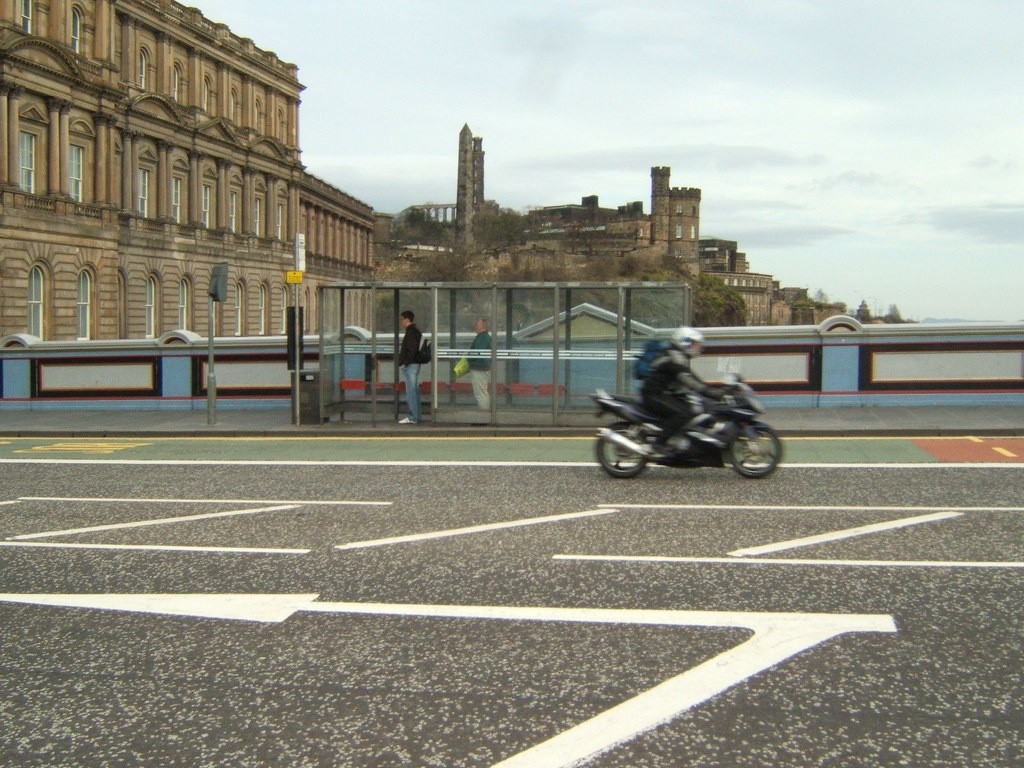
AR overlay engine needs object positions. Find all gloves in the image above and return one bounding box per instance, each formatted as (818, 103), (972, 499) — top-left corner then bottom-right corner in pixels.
(701, 386), (725, 401)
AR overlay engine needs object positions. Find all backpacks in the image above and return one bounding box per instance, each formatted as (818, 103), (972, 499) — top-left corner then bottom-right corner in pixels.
(410, 328), (431, 364)
(633, 339), (677, 379)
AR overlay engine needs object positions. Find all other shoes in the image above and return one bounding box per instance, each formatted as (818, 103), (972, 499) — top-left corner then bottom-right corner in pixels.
(652, 440), (675, 455)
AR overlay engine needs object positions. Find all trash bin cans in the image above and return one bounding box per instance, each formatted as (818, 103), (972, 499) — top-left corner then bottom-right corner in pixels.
(289, 368), (330, 426)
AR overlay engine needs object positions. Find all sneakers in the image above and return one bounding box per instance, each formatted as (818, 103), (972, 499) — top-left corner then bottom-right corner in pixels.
(398, 418), (418, 423)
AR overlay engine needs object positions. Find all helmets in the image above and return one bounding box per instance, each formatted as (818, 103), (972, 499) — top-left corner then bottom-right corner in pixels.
(670, 325), (706, 359)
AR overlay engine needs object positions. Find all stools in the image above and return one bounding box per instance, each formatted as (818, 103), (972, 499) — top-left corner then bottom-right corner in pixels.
(340, 379), (564, 396)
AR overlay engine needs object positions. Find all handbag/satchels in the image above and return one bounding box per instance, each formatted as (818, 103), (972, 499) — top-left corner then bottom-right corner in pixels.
(454, 357), (470, 377)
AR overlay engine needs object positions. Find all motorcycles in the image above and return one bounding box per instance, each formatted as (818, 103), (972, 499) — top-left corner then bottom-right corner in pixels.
(586, 373), (783, 479)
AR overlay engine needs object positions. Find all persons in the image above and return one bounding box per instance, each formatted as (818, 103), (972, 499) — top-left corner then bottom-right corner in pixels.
(467, 319), (492, 411)
(398, 311), (422, 424)
(640, 326), (725, 455)
